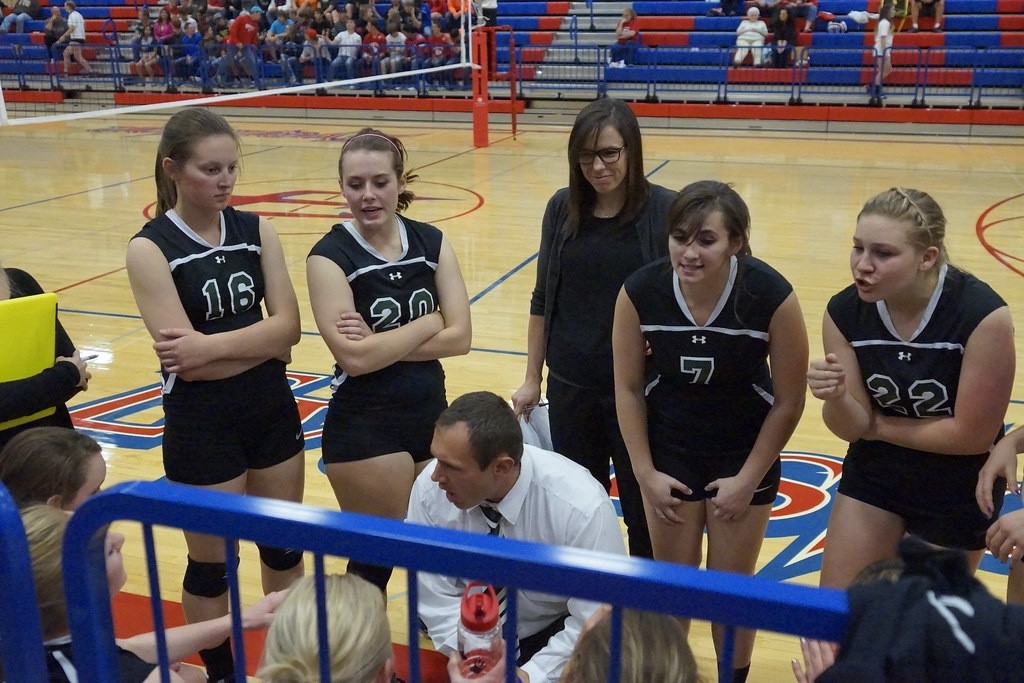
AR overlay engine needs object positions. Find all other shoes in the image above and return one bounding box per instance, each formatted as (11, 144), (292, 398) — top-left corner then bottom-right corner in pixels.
(609, 59), (625, 68)
(907, 27), (919, 32)
(932, 26), (943, 32)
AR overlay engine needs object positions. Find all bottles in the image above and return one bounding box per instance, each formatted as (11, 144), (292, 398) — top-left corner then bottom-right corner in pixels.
(455, 578), (504, 681)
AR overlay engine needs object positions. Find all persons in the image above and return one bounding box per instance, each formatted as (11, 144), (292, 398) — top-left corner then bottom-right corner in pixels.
(910, 0), (945, 32)
(125, 109), (305, 683)
(609, 9), (639, 69)
(793, 549), (969, 683)
(711, 0), (817, 68)
(512, 98), (682, 556)
(305, 127), (471, 641)
(610, 180), (810, 683)
(805, 186), (1014, 591)
(559, 606), (706, 683)
(974, 424), (1024, 608)
(0, 262), (93, 430)
(1, 1), (501, 97)
(866, 4), (898, 108)
(404, 390), (627, 683)
(0, 427), (396, 683)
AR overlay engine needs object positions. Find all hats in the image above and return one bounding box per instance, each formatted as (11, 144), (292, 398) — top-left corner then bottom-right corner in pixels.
(305, 29), (316, 38)
(251, 6), (265, 13)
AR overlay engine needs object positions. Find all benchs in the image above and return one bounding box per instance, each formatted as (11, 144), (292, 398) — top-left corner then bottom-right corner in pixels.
(0, 0), (1024, 126)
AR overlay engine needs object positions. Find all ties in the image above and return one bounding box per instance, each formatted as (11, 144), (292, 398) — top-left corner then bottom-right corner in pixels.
(479, 505), (520, 662)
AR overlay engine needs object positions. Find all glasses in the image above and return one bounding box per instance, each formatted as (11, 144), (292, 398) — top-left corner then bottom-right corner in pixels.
(576, 144), (626, 164)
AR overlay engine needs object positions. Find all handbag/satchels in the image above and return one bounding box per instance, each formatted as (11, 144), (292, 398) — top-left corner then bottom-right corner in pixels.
(827, 20), (848, 34)
(818, 10), (834, 21)
